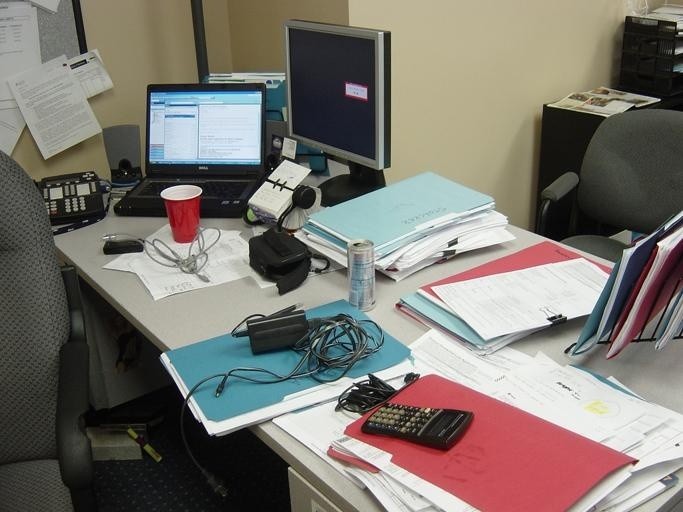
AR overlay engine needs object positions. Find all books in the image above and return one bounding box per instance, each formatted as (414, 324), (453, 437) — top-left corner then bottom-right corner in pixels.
(161, 298), (415, 436)
(397, 207), (683, 357)
(545, 85), (661, 117)
(296, 172), (517, 281)
(631, 5), (682, 73)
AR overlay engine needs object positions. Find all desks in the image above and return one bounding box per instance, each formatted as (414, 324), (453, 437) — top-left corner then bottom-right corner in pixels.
(54, 224), (682, 511)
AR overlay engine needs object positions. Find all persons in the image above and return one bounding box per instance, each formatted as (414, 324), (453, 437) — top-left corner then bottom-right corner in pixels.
(111, 311), (144, 375)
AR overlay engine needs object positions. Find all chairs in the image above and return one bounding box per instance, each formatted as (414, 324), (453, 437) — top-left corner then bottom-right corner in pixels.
(0, 151), (100, 512)
(535, 109), (682, 261)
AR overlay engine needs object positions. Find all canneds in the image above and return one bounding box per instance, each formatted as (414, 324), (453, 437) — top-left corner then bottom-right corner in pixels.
(346, 239), (376, 312)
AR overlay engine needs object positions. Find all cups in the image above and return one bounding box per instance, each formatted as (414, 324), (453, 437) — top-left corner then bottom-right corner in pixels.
(160, 184), (202, 243)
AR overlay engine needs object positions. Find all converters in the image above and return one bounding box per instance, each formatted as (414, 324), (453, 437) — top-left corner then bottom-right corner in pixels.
(246, 310), (308, 355)
(103, 240), (143, 254)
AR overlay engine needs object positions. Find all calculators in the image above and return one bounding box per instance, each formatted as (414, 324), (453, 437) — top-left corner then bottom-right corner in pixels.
(361, 402), (475, 451)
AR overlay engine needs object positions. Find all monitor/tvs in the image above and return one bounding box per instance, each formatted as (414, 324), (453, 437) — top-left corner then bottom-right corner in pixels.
(282, 19), (392, 207)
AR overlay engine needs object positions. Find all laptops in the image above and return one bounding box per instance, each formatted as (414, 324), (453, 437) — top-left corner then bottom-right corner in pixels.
(114, 82), (267, 216)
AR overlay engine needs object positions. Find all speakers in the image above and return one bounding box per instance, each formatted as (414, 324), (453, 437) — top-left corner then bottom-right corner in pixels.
(102, 124), (143, 186)
(266, 119), (287, 168)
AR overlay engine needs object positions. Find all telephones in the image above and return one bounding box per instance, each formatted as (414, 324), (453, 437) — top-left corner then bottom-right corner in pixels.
(32, 171), (106, 225)
